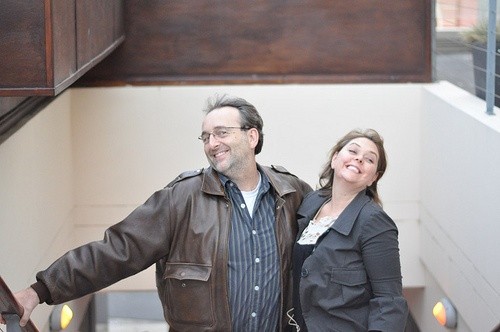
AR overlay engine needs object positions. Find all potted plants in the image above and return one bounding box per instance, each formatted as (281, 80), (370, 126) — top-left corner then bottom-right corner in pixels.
(463, 18), (500, 107)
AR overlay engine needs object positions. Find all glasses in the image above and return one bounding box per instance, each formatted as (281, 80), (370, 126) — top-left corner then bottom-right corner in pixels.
(199, 126), (251, 143)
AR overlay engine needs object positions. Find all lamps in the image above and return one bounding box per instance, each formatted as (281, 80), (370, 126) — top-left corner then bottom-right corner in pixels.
(49, 304), (73, 332)
(433, 298), (456, 328)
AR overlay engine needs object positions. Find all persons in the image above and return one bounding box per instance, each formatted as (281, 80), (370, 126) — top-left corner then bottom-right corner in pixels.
(292, 129), (407, 332)
(0, 95), (314, 332)
